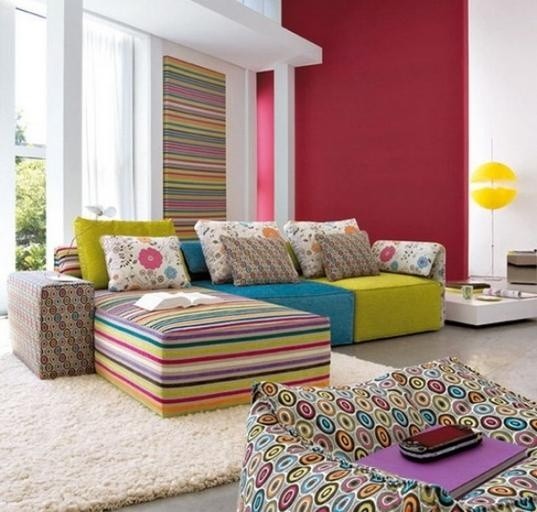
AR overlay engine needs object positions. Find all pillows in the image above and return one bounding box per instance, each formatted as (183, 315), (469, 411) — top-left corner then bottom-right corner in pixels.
(97, 235), (194, 289)
(219, 234), (299, 286)
(193, 219), (298, 285)
(283, 217), (366, 277)
(74, 219), (179, 288)
(314, 230), (382, 282)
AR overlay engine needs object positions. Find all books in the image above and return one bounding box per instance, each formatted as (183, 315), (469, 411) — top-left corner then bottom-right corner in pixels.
(482, 288), (536, 299)
(352, 423), (530, 501)
(132, 291), (224, 311)
(445, 282), (490, 289)
(444, 287), (487, 294)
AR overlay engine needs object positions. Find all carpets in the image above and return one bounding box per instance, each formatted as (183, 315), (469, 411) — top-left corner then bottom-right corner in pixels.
(0, 316), (401, 512)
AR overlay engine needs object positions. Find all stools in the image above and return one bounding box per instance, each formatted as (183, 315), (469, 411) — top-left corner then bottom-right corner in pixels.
(234, 356), (536, 512)
(6, 269), (96, 380)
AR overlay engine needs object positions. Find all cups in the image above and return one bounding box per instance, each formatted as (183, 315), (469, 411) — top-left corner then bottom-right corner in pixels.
(461, 285), (474, 301)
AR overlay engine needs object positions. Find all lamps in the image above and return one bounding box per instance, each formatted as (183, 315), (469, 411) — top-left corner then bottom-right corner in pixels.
(468, 160), (518, 282)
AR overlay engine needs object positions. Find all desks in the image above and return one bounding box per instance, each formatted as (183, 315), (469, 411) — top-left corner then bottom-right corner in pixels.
(506, 250), (536, 285)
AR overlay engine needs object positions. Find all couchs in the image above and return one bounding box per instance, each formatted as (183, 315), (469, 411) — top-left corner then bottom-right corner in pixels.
(54, 240), (446, 416)
